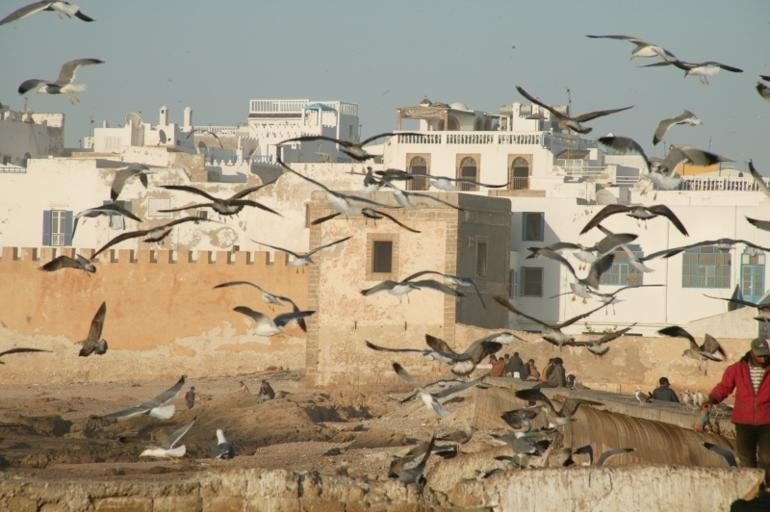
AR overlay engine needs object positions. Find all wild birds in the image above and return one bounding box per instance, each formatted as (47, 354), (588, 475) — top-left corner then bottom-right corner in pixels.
(585, 33), (685, 69)
(0, 0), (96, 27)
(18, 58), (105, 105)
(755, 75), (770, 100)
(1, 347), (51, 365)
(35, 85), (770, 486)
(636, 59), (744, 87)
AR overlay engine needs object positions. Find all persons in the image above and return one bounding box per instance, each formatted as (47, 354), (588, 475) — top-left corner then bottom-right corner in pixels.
(730, 434), (769, 511)
(489, 352), (576, 386)
(185, 386), (195, 408)
(239, 381), (250, 392)
(652, 377), (679, 402)
(701, 337), (770, 490)
(257, 379), (275, 404)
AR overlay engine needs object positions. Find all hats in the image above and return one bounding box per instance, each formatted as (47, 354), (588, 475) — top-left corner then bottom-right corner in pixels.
(750, 336), (770, 357)
(659, 376), (671, 385)
